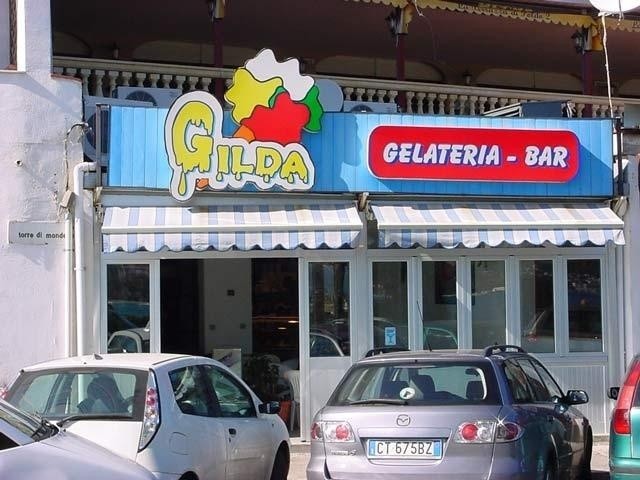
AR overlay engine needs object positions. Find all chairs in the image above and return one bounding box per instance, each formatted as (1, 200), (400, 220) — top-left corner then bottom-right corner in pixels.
(385, 375), (485, 401)
(283, 369), (301, 434)
(80, 378), (126, 413)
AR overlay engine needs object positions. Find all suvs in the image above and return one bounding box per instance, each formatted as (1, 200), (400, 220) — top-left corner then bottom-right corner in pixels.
(296, 343), (599, 480)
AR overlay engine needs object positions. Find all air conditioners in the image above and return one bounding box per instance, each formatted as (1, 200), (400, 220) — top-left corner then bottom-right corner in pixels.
(343, 101), (397, 114)
(83, 96), (153, 169)
(111, 86), (182, 109)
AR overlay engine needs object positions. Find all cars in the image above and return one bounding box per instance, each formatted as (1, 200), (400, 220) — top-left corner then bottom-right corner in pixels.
(0, 352), (294, 479)
(0, 393), (159, 480)
(602, 346), (640, 480)
